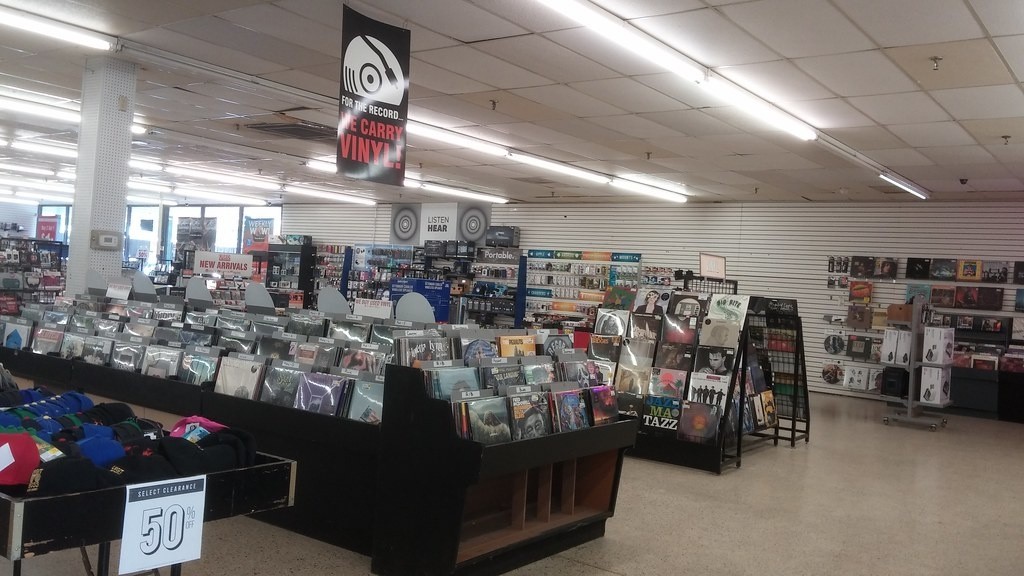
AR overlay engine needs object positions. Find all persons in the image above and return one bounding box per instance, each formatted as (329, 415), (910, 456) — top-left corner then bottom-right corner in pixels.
(519, 406), (549, 440)
(595, 366), (604, 383)
(872, 257), (896, 279)
(697, 348), (732, 377)
(715, 389), (726, 405)
(695, 385), (703, 403)
(708, 385), (717, 404)
(702, 385), (709, 403)
(634, 290), (664, 315)
(601, 313), (619, 336)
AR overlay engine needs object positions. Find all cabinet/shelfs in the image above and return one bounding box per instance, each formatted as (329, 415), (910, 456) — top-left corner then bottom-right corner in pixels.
(881, 294), (1024, 432)
(370, 363), (637, 576)
(0, 237), (69, 317)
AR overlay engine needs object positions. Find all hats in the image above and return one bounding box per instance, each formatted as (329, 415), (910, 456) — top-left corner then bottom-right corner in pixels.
(0, 363), (260, 498)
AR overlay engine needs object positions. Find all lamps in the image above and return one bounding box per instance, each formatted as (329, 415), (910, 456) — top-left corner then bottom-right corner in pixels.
(0, 0), (930, 205)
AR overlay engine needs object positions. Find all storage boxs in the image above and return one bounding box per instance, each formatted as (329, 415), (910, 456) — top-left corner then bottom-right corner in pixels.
(922, 327), (954, 364)
(887, 304), (913, 321)
(895, 330), (913, 365)
(881, 330), (899, 365)
(919, 366), (951, 404)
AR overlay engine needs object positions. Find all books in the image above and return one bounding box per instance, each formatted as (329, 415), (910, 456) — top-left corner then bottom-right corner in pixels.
(2, 248), (415, 423)
(586, 285), (808, 444)
(841, 254), (1024, 392)
(398, 334), (627, 444)
